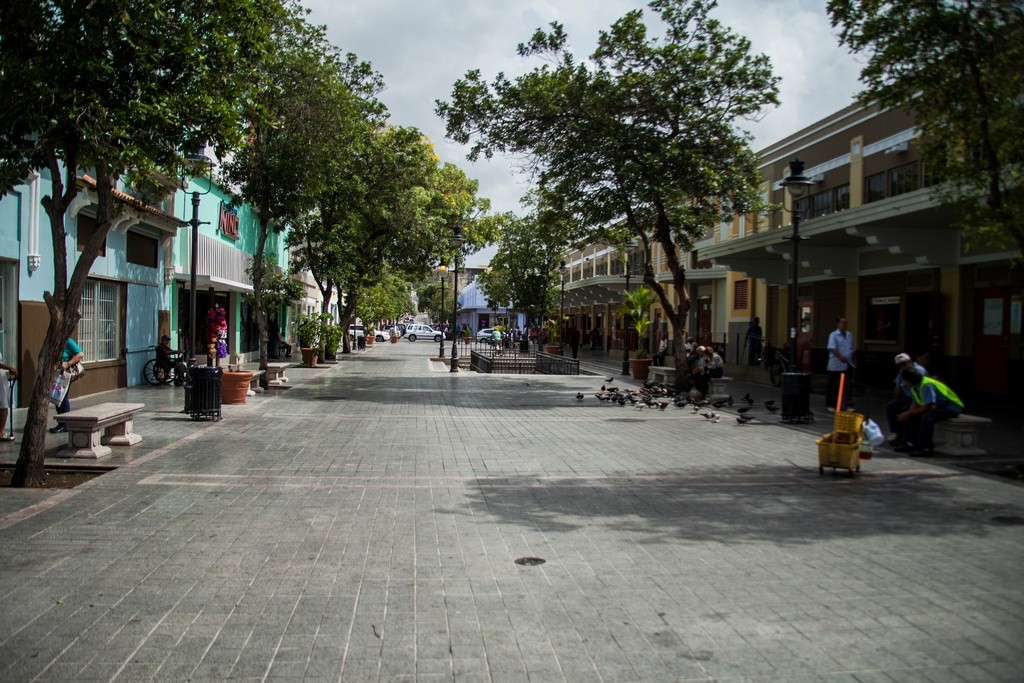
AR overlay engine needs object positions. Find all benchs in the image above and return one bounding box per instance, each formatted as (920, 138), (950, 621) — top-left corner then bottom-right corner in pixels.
(708, 376), (733, 401)
(52, 402), (146, 459)
(934, 414), (993, 457)
(646, 367), (676, 387)
(246, 369), (266, 396)
(260, 362), (291, 385)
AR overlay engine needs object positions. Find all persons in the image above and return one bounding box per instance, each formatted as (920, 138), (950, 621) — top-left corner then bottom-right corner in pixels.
(156, 335), (188, 384)
(887, 352), (933, 444)
(744, 321), (758, 365)
(430, 321), (548, 352)
(743, 317), (764, 365)
(297, 317), (313, 359)
(568, 326), (580, 358)
(278, 332), (292, 357)
(0, 351), (17, 440)
(822, 318), (856, 411)
(54, 335), (84, 435)
(896, 367), (965, 455)
(652, 332), (724, 392)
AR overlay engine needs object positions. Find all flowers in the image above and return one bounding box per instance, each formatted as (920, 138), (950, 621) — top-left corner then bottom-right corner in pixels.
(207, 304), (227, 366)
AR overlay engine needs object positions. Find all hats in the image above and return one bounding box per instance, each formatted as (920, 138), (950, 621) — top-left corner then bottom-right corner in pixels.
(894, 353), (911, 364)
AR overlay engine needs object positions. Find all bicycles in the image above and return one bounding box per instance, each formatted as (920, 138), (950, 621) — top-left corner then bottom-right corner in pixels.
(767, 343), (806, 388)
(488, 335), (503, 357)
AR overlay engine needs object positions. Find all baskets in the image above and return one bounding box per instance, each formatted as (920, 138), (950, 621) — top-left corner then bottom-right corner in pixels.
(819, 411), (866, 474)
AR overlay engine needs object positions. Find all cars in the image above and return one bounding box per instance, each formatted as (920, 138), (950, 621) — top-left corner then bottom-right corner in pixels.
(475, 328), (509, 343)
(347, 317), (414, 343)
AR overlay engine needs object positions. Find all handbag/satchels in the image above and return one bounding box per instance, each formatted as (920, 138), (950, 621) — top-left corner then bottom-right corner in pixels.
(62, 361), (87, 382)
(50, 372), (71, 408)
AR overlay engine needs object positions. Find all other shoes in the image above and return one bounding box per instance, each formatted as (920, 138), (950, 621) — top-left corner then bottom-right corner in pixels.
(888, 434), (935, 459)
(1, 434), (17, 441)
(50, 424), (68, 433)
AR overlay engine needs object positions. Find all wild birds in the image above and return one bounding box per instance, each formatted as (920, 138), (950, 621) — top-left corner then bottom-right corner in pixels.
(576, 377), (777, 425)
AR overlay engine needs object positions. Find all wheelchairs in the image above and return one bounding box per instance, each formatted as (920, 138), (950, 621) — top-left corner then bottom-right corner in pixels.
(142, 344), (190, 386)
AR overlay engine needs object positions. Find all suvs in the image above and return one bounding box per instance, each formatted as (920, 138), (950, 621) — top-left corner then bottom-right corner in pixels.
(405, 324), (447, 343)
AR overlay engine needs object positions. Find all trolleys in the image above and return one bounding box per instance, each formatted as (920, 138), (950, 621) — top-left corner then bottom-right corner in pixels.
(816, 371), (865, 474)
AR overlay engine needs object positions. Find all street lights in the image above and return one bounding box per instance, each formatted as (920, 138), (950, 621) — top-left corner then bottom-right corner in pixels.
(556, 260), (568, 358)
(435, 256), (449, 357)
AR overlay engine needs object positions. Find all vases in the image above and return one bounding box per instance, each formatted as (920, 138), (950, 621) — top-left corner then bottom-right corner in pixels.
(220, 369), (252, 404)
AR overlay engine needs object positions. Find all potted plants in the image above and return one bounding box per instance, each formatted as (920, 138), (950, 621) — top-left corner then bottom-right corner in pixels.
(614, 285), (658, 379)
(462, 326), (472, 345)
(367, 325), (376, 344)
(390, 331), (398, 343)
(546, 317), (564, 355)
(290, 313), (322, 365)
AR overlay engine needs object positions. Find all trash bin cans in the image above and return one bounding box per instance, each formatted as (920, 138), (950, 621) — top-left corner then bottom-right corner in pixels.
(781, 372), (814, 424)
(189, 366), (223, 422)
(520, 340), (528, 353)
(357, 336), (365, 351)
